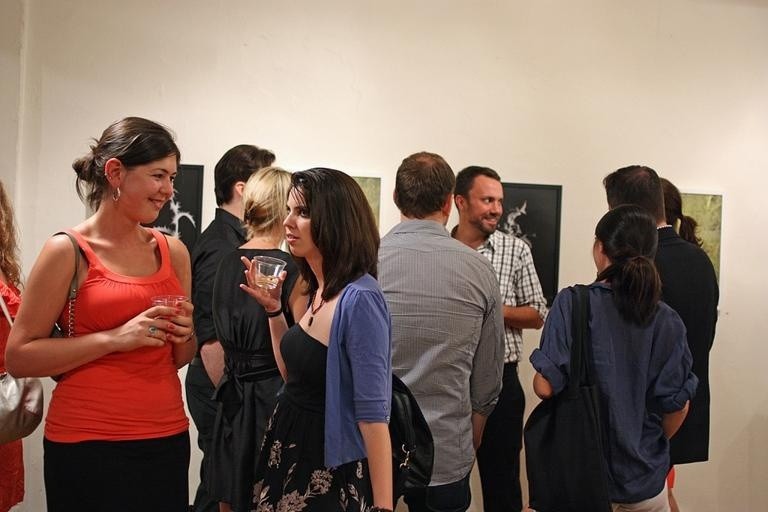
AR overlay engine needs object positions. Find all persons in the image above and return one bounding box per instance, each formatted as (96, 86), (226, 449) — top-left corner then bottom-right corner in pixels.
(449, 163), (552, 510)
(528, 203), (701, 512)
(664, 178), (706, 512)
(376, 148), (504, 512)
(207, 165), (289, 508)
(1, 183), (43, 509)
(240, 165), (400, 512)
(5, 116), (199, 509)
(185, 141), (275, 451)
(600, 164), (718, 467)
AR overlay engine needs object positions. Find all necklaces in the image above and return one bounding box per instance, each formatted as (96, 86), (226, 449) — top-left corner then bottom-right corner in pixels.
(308, 287), (324, 328)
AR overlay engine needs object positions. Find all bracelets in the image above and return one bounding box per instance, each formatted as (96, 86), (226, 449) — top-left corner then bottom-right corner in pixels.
(185, 327), (196, 342)
(264, 306), (285, 318)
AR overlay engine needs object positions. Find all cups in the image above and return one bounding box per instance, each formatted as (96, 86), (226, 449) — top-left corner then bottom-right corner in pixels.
(149, 294), (191, 318)
(252, 254), (287, 290)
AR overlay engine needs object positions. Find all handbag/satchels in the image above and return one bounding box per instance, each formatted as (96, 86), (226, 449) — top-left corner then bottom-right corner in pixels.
(1, 373), (43, 445)
(48, 324), (65, 383)
(524, 385), (613, 511)
(389, 374), (433, 488)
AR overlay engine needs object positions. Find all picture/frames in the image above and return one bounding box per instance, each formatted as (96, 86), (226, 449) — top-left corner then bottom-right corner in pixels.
(495, 181), (563, 308)
(140, 164), (204, 255)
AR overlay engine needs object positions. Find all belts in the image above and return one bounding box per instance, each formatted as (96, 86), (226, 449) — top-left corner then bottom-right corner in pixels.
(190, 358), (205, 368)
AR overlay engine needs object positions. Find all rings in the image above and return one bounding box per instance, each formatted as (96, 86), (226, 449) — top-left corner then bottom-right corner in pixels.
(148, 325), (156, 336)
